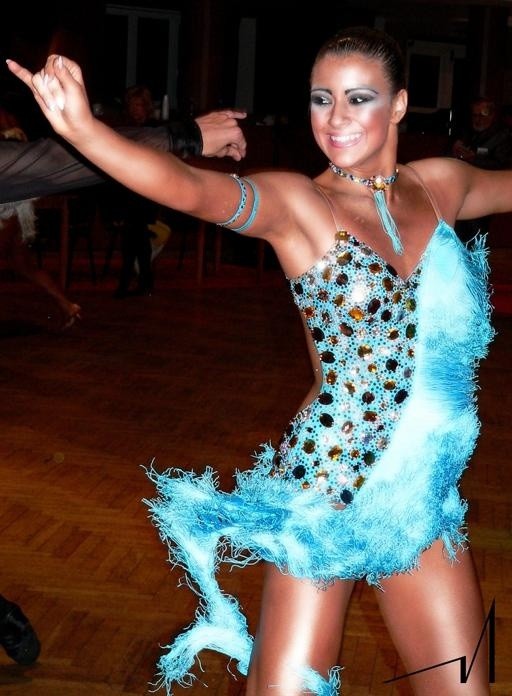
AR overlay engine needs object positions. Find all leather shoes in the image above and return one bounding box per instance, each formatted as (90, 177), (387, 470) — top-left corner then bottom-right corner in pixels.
(0, 591), (44, 669)
(55, 304), (85, 333)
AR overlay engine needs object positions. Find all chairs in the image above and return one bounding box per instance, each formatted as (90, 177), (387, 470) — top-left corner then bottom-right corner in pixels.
(25, 179), (218, 288)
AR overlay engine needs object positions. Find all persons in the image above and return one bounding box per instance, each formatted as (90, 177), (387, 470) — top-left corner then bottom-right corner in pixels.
(0, 0), (512, 696)
